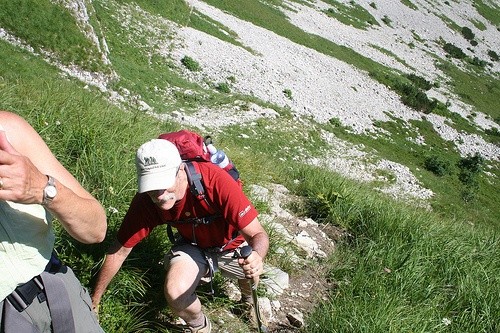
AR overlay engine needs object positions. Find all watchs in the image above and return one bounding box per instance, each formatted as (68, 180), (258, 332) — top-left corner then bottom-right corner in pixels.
(40, 175), (57, 209)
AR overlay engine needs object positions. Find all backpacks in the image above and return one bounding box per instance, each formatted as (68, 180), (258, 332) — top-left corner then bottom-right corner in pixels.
(158, 131), (243, 214)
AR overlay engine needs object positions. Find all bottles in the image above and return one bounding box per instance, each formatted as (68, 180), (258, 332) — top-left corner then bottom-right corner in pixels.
(206, 144), (239, 179)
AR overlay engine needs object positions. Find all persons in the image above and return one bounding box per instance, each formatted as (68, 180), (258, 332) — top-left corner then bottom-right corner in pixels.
(91, 139), (269, 333)
(0, 111), (108, 333)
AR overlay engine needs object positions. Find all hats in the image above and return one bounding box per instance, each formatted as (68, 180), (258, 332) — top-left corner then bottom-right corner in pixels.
(135, 139), (182, 193)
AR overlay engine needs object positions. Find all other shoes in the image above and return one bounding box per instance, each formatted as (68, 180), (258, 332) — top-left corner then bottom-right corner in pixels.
(237, 300), (267, 329)
(182, 315), (211, 333)
